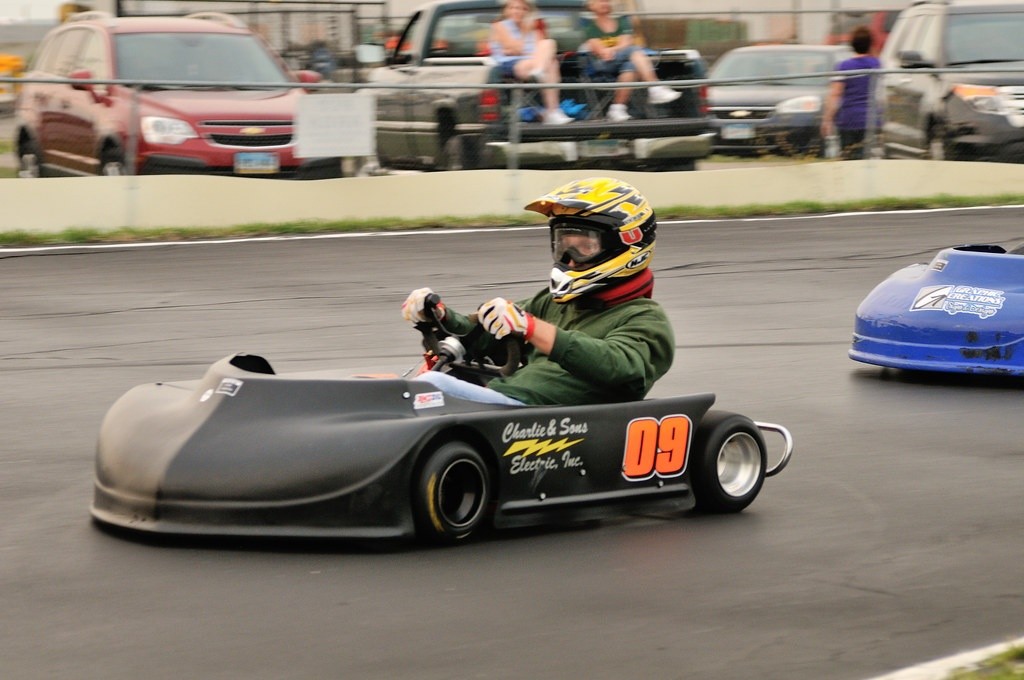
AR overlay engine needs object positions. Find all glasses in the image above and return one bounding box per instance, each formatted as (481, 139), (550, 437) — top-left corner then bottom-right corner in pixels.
(553, 222), (620, 265)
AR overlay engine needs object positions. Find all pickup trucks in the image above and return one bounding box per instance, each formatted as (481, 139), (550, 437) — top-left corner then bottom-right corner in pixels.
(355, 0), (714, 172)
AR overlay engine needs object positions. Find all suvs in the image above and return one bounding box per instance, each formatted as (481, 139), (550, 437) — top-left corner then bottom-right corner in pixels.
(12, 11), (323, 180)
(873, 1), (1023, 165)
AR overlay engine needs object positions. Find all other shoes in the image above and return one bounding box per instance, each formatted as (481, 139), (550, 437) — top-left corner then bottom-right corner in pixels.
(606, 104), (634, 122)
(648, 86), (682, 103)
(543, 108), (576, 125)
(529, 68), (546, 82)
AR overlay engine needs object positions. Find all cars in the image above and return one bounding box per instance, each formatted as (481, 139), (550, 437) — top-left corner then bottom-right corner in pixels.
(704, 45), (857, 156)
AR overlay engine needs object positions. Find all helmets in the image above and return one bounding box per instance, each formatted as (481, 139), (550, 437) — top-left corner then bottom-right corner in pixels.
(523, 177), (658, 304)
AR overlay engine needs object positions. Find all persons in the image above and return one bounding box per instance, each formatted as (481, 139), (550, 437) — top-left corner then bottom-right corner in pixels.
(578, 0), (683, 121)
(487, 1), (576, 127)
(822, 27), (887, 159)
(399, 176), (675, 404)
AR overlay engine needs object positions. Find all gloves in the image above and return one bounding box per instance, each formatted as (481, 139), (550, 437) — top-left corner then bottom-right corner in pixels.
(402, 287), (446, 324)
(477, 297), (535, 343)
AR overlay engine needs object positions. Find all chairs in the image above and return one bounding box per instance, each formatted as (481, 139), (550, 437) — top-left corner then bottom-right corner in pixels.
(487, 17), (560, 124)
(575, 14), (670, 121)
